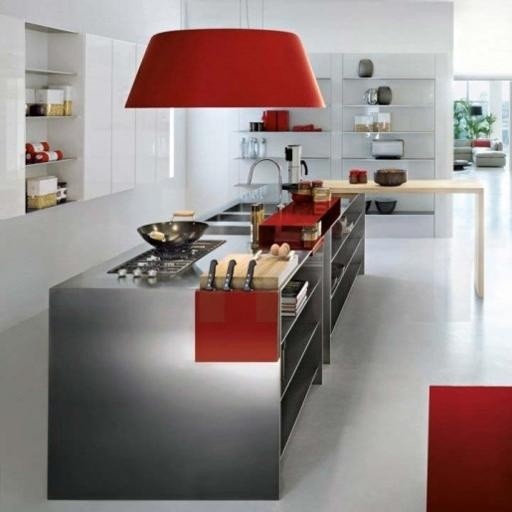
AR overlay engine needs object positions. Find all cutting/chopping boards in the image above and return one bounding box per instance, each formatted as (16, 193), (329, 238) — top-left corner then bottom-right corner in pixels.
(200, 253), (299, 289)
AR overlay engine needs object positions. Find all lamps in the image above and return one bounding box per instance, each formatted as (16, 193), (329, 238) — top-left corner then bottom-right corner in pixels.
(121, 1), (328, 113)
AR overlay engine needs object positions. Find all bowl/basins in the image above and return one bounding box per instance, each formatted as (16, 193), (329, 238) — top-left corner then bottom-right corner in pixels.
(373, 169), (408, 187)
(374, 200), (397, 215)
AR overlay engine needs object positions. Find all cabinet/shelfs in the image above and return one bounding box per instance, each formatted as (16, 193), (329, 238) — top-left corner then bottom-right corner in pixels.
(230, 75), (438, 221)
(281, 190), (365, 499)
(23, 20), (85, 217)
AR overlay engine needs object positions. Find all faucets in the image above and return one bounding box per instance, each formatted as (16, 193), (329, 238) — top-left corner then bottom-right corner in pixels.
(246, 157), (283, 201)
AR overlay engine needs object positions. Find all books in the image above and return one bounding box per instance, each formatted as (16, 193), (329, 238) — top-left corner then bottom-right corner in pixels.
(281, 278), (310, 317)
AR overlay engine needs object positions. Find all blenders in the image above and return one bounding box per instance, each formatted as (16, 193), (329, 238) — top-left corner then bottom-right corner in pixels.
(284, 144), (308, 183)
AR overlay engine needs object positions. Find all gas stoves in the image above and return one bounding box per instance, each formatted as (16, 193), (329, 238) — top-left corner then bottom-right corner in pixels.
(107, 238), (227, 277)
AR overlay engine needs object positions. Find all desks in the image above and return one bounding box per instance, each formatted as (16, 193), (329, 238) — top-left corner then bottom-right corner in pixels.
(287, 180), (489, 299)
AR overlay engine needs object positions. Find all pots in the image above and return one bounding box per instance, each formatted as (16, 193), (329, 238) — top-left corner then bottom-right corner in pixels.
(137, 211), (210, 250)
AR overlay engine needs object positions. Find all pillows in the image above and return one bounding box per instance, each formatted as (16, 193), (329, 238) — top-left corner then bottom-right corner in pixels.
(471, 139), (497, 150)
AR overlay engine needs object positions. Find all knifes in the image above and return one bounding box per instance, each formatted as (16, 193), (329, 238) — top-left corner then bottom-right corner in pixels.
(206, 260), (256, 291)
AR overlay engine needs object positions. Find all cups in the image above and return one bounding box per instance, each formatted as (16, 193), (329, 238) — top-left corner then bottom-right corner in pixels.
(257, 122), (264, 131)
(249, 122), (258, 132)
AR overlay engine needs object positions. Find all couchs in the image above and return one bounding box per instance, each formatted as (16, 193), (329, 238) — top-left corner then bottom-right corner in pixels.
(454, 137), (506, 169)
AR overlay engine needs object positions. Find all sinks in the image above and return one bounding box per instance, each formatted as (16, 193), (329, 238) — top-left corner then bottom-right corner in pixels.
(222, 201), (280, 213)
(205, 212), (272, 223)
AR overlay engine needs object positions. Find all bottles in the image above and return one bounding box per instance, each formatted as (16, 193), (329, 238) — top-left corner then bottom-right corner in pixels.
(240, 138), (268, 159)
(250, 202), (265, 251)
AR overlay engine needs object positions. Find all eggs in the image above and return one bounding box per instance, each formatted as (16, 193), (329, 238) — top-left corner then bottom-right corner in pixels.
(271, 242), (290, 255)
